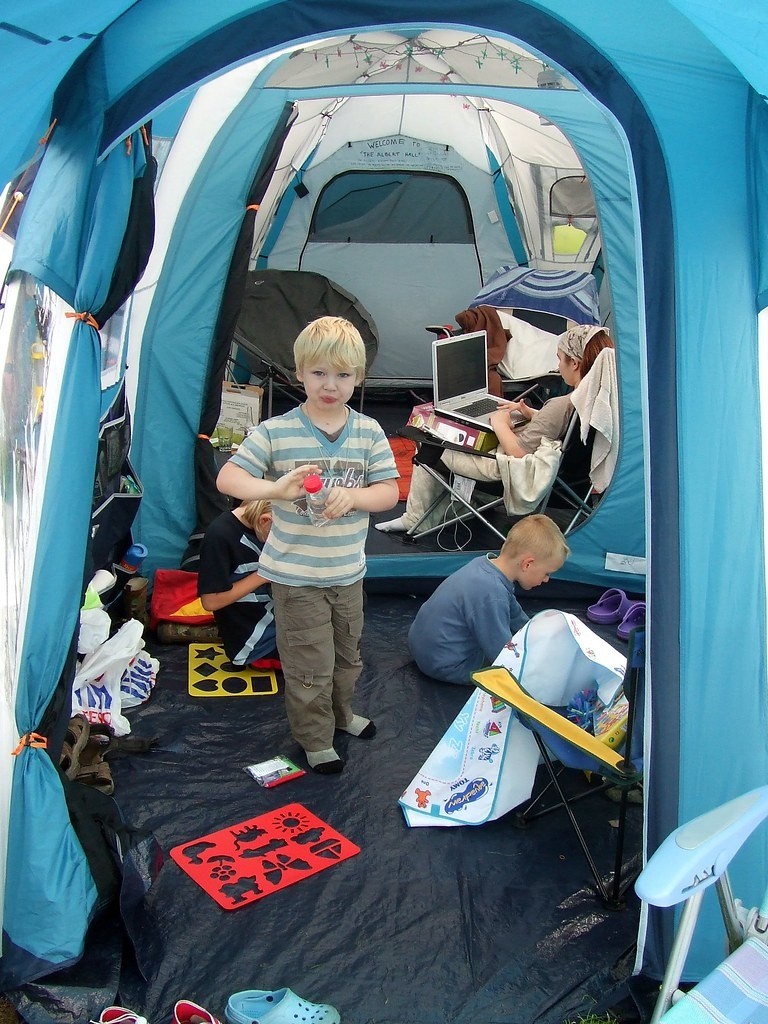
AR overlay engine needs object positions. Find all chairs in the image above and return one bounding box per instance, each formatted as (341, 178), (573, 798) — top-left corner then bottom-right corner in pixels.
(225, 268), (379, 419)
(468, 626), (645, 910)
(425, 265), (602, 403)
(634, 785), (768, 1024)
(402, 347), (620, 545)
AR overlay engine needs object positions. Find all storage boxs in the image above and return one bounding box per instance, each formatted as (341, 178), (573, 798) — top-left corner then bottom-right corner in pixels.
(406, 401), (499, 453)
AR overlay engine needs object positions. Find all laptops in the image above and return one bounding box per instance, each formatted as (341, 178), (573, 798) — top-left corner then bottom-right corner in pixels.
(432, 329), (539, 434)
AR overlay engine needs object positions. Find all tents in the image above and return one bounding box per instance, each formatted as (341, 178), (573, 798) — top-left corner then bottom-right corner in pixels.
(94, 24), (651, 600)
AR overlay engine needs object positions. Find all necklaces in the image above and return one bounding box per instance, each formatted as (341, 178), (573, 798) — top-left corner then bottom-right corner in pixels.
(305, 398), (350, 487)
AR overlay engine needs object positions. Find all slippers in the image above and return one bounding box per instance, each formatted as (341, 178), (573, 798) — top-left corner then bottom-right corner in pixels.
(224, 988), (341, 1024)
(616, 603), (646, 640)
(586, 588), (645, 625)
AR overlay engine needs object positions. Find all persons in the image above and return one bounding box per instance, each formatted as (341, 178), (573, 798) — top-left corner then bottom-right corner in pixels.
(407, 513), (570, 685)
(216, 316), (399, 771)
(196, 498), (281, 666)
(374, 325), (614, 536)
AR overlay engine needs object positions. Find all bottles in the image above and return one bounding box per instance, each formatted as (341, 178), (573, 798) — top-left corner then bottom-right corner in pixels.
(303, 475), (334, 527)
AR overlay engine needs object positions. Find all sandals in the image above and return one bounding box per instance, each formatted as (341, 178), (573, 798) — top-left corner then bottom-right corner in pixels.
(59, 714), (90, 782)
(80, 722), (115, 796)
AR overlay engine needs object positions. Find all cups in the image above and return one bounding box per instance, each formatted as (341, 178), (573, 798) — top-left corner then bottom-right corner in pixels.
(217, 426), (233, 452)
